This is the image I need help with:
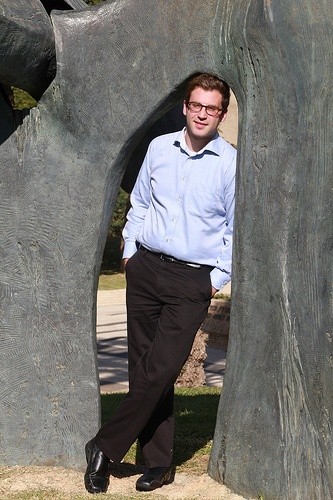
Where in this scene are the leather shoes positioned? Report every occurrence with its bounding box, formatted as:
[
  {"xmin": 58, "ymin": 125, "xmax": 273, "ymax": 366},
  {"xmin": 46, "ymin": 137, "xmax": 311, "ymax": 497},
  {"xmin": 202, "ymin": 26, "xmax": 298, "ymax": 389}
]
[
  {"xmin": 84, "ymin": 437, "xmax": 109, "ymax": 493},
  {"xmin": 136, "ymin": 465, "xmax": 175, "ymax": 491}
]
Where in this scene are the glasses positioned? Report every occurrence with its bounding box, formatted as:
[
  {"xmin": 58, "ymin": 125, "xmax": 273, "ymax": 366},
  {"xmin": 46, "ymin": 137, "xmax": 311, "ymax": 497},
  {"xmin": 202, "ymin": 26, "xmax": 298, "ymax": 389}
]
[{"xmin": 186, "ymin": 101, "xmax": 223, "ymax": 116}]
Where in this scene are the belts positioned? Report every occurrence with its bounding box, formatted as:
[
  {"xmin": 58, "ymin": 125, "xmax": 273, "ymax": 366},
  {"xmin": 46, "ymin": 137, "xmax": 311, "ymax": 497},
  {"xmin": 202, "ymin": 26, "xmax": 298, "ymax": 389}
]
[{"xmin": 137, "ymin": 241, "xmax": 201, "ymax": 269}]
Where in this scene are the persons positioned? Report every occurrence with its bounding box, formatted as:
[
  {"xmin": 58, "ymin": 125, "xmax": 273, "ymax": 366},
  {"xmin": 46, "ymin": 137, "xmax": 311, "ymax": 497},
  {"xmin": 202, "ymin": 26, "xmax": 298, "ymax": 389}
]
[{"xmin": 84, "ymin": 73, "xmax": 236, "ymax": 493}]
